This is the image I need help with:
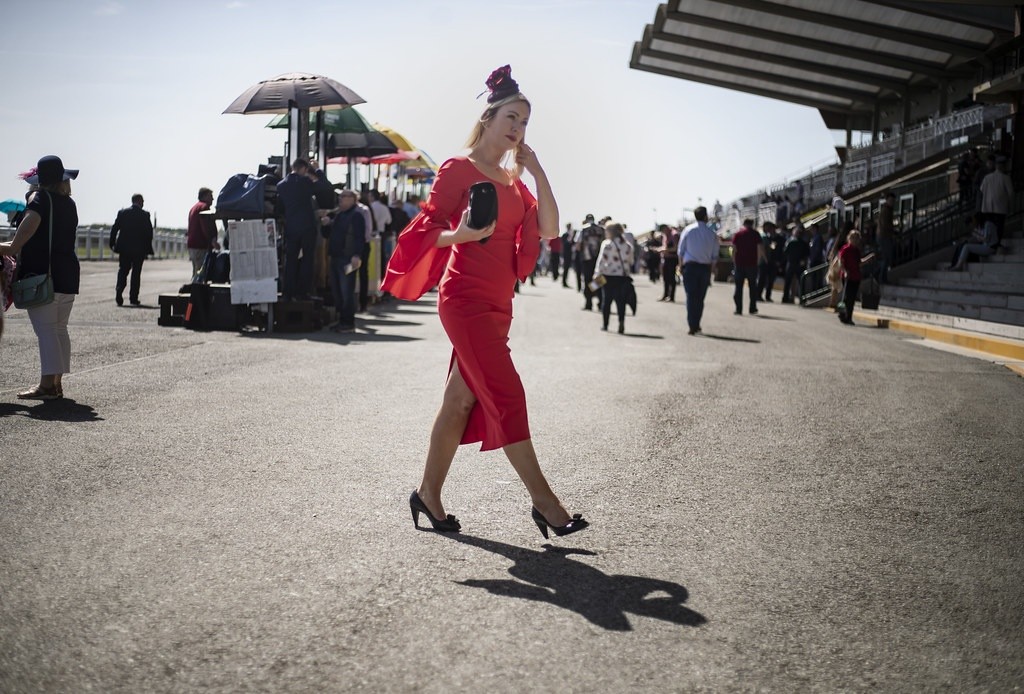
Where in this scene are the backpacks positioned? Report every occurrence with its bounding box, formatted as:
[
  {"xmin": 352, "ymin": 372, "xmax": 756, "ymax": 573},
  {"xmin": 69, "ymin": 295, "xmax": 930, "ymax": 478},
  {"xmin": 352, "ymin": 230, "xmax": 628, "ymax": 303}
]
[
  {"xmin": 216, "ymin": 173, "xmax": 265, "ymax": 214},
  {"xmin": 202, "ymin": 249, "xmax": 230, "ymax": 283}
]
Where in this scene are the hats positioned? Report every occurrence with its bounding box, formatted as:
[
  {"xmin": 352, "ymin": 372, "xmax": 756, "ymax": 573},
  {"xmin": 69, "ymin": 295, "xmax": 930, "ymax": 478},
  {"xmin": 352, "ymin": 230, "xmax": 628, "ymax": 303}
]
[
  {"xmin": 17, "ymin": 155, "xmax": 79, "ymax": 185},
  {"xmin": 582, "ymin": 214, "xmax": 594, "ymax": 225},
  {"xmin": 484, "ymin": 64, "xmax": 528, "ymax": 109}
]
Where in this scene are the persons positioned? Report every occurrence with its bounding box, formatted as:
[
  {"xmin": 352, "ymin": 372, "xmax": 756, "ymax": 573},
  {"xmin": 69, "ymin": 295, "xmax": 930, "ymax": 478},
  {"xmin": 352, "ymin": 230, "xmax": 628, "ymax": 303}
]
[
  {"xmin": 678, "ymin": 205, "xmax": 719, "ymax": 335},
  {"xmin": 732, "ymin": 218, "xmax": 763, "ymax": 317},
  {"xmin": 0, "ymin": 155, "xmax": 80, "ymax": 399},
  {"xmin": 321, "ymin": 190, "xmax": 422, "ymax": 332},
  {"xmin": 620, "ymin": 224, "xmax": 683, "ymax": 303},
  {"xmin": 110, "ymin": 195, "xmax": 154, "ymax": 306},
  {"xmin": 948, "ymin": 147, "xmax": 1012, "ymax": 271},
  {"xmin": 593, "ymin": 220, "xmax": 634, "ymax": 334},
  {"xmin": 514, "ymin": 215, "xmax": 611, "ymax": 310},
  {"xmin": 877, "ymin": 191, "xmax": 899, "ymax": 285},
  {"xmin": 188, "ymin": 187, "xmax": 221, "ymax": 285},
  {"xmin": 380, "ymin": 63, "xmax": 590, "ymax": 539},
  {"xmin": 757, "ymin": 180, "xmax": 845, "ymax": 306},
  {"xmin": 274, "ymin": 160, "xmax": 336, "ymax": 303},
  {"xmin": 838, "ymin": 229, "xmax": 875, "ymax": 325},
  {"xmin": 222, "ymin": 226, "xmax": 229, "ymax": 250}
]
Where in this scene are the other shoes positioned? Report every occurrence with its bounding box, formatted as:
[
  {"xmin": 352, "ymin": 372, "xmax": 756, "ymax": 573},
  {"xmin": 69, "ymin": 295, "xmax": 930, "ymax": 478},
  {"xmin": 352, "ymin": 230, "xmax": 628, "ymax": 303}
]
[
  {"xmin": 56, "ymin": 383, "xmax": 63, "ymax": 397},
  {"xmin": 130, "ymin": 300, "xmax": 140, "ymax": 305},
  {"xmin": 116, "ymin": 287, "xmax": 124, "ymax": 305},
  {"xmin": 766, "ymin": 298, "xmax": 772, "ymax": 302},
  {"xmin": 15, "ymin": 384, "xmax": 58, "ymax": 400},
  {"xmin": 756, "ymin": 297, "xmax": 764, "ymax": 302},
  {"xmin": 330, "ymin": 320, "xmax": 356, "ymax": 335}
]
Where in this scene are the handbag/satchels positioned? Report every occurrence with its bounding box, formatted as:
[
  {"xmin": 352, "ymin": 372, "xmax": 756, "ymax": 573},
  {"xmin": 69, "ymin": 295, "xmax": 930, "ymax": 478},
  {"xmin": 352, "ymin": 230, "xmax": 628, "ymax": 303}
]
[{"xmin": 11, "ymin": 273, "xmax": 55, "ymax": 309}]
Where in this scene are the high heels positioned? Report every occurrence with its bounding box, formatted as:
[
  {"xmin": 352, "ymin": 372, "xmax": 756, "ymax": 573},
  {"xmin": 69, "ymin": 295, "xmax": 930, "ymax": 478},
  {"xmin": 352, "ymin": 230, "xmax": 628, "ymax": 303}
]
[
  {"xmin": 531, "ymin": 504, "xmax": 590, "ymax": 540},
  {"xmin": 409, "ymin": 489, "xmax": 462, "ymax": 532}
]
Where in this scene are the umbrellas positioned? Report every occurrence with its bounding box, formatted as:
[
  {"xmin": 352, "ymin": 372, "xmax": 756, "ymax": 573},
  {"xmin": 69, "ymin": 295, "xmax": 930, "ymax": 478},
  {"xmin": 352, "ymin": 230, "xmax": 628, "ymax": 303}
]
[{"xmin": 223, "ymin": 72, "xmax": 437, "ymax": 203}]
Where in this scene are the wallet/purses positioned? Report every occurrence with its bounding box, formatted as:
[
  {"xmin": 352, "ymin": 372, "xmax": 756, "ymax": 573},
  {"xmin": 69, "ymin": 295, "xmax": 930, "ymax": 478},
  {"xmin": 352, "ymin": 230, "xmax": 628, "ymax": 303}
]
[{"xmin": 467, "ymin": 181, "xmax": 498, "ymax": 243}]
